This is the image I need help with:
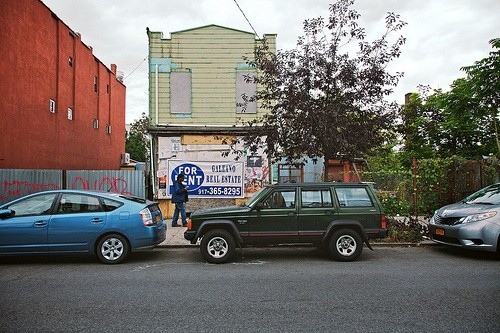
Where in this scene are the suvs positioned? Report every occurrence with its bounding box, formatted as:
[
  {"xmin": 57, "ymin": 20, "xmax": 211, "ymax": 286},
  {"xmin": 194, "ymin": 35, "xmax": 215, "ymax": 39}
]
[{"xmin": 184, "ymin": 180, "xmax": 389, "ymax": 265}]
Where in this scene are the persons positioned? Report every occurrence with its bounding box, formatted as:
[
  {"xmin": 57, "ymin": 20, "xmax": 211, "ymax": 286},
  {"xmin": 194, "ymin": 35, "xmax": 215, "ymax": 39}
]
[{"xmin": 169, "ymin": 173, "xmax": 190, "ymax": 228}]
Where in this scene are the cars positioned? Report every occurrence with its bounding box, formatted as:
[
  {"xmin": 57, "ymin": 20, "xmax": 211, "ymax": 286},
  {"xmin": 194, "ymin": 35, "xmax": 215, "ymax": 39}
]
[
  {"xmin": 429, "ymin": 182, "xmax": 500, "ymax": 254},
  {"xmin": 0, "ymin": 190, "xmax": 168, "ymax": 264}
]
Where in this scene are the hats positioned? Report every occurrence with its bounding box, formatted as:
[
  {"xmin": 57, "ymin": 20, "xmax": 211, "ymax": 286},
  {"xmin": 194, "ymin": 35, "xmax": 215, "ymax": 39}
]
[{"xmin": 178, "ymin": 174, "xmax": 185, "ymax": 178}]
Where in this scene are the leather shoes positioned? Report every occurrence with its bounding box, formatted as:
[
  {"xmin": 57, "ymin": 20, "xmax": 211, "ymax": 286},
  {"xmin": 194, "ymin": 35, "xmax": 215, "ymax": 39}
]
[{"xmin": 172, "ymin": 223, "xmax": 188, "ymax": 227}]
